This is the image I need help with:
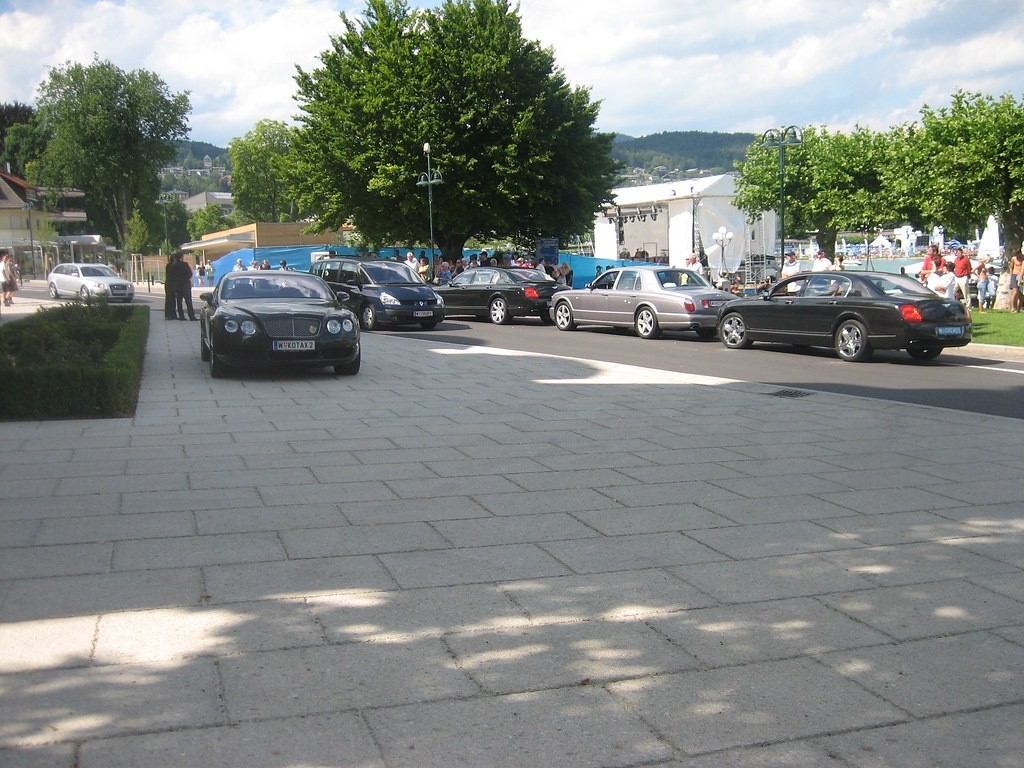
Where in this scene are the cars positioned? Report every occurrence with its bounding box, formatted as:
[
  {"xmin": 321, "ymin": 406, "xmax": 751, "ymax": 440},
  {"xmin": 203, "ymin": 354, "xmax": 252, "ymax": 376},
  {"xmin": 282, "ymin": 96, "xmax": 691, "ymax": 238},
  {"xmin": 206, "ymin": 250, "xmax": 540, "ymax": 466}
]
[
  {"xmin": 714, "ymin": 269, "xmax": 973, "ymax": 364},
  {"xmin": 430, "ymin": 265, "xmax": 572, "ymax": 325},
  {"xmin": 549, "ymin": 266, "xmax": 742, "ymax": 340},
  {"xmin": 48, "ymin": 262, "xmax": 136, "ymax": 303},
  {"xmin": 955, "ymin": 256, "xmax": 1002, "ymax": 301},
  {"xmin": 199, "ymin": 269, "xmax": 361, "ymax": 379}
]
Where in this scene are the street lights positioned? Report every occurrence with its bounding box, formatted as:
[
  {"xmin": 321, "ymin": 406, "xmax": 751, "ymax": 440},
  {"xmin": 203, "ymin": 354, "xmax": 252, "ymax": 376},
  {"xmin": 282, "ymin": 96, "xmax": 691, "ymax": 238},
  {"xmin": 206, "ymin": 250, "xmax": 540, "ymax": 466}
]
[
  {"xmin": 712, "ymin": 226, "xmax": 734, "ymax": 276},
  {"xmin": 23, "ymin": 201, "xmax": 37, "ymax": 278},
  {"xmin": 416, "ymin": 141, "xmax": 446, "ymax": 280},
  {"xmin": 760, "ymin": 125, "xmax": 803, "ymax": 274},
  {"xmin": 155, "ymin": 193, "xmax": 174, "ymax": 262}
]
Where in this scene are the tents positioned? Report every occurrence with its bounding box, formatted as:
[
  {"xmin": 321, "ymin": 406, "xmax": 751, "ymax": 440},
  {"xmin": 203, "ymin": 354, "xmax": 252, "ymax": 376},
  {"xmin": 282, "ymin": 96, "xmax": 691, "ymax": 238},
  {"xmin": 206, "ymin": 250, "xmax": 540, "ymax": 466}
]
[{"xmin": 593, "ymin": 174, "xmax": 775, "ymax": 273}]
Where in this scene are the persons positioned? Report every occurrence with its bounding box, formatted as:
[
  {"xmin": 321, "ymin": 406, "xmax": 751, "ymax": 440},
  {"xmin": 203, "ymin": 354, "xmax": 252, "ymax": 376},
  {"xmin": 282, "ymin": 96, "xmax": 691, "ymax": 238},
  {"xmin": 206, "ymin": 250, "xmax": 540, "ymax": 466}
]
[
  {"xmin": 535, "ymin": 257, "xmax": 574, "ymax": 289},
  {"xmin": 1010, "ymin": 247, "xmax": 1024, "ymax": 313},
  {"xmin": 433, "ymin": 254, "xmax": 469, "ymax": 287},
  {"xmin": 830, "ymin": 255, "xmax": 844, "ymax": 296},
  {"xmin": 813, "ymin": 249, "xmax": 832, "ymax": 271},
  {"xmin": 279, "ymin": 260, "xmax": 288, "ymax": 271},
  {"xmin": 954, "ymin": 247, "xmax": 972, "ymax": 310},
  {"xmin": 232, "ymin": 259, "xmax": 271, "ymax": 272},
  {"xmin": 164, "ymin": 252, "xmax": 201, "ymax": 321},
  {"xmin": 781, "ymin": 252, "xmax": 805, "ymax": 296},
  {"xmin": 595, "ymin": 265, "xmax": 603, "ymax": 278},
  {"xmin": 971, "ymin": 258, "xmax": 998, "ymax": 315},
  {"xmin": 468, "ymin": 250, "xmax": 539, "ymax": 269},
  {"xmin": 606, "ymin": 265, "xmax": 618, "ymax": 280},
  {"xmin": 918, "ymin": 243, "xmax": 956, "ymax": 299},
  {"xmin": 685, "ymin": 253, "xmax": 707, "ymax": 277},
  {"xmin": 390, "ymin": 249, "xmax": 432, "ymax": 283},
  {"xmin": 196, "ymin": 259, "xmax": 216, "ymax": 287},
  {"xmin": 0, "ymin": 253, "xmax": 18, "ymax": 306}
]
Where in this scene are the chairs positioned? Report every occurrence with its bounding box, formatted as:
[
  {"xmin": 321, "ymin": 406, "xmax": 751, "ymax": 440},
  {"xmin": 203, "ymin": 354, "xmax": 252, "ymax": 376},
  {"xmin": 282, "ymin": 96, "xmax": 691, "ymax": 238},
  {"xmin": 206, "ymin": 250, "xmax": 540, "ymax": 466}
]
[{"xmin": 234, "ymin": 283, "xmax": 254, "ymax": 299}]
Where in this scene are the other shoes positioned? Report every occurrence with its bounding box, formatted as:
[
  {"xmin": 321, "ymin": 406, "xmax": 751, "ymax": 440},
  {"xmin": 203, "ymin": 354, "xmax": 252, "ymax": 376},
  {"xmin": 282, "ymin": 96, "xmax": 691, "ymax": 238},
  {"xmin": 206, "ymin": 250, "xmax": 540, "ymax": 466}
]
[
  {"xmin": 3, "ymin": 297, "xmax": 14, "ymax": 306},
  {"xmin": 165, "ymin": 315, "xmax": 200, "ymax": 321}
]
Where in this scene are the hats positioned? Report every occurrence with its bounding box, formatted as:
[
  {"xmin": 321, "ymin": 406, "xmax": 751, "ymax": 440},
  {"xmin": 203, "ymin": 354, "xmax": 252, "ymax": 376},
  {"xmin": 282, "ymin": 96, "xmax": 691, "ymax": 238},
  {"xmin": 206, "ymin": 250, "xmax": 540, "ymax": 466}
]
[
  {"xmin": 479, "ymin": 251, "xmax": 487, "ymax": 255},
  {"xmin": 818, "ymin": 250, "xmax": 825, "ymax": 253},
  {"xmin": 785, "ymin": 251, "xmax": 795, "ymax": 256}
]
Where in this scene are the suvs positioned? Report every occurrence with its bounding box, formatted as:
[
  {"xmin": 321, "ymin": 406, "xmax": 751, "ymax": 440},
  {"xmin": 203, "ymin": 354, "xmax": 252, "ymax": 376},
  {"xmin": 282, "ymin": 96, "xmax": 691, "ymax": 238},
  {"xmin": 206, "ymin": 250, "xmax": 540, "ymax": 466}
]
[{"xmin": 307, "ymin": 254, "xmax": 447, "ymax": 331}]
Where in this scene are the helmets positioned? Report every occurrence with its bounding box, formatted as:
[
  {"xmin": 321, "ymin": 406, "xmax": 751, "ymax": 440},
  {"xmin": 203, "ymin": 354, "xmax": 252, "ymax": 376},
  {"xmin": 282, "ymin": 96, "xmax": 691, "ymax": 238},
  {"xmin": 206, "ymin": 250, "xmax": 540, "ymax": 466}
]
[{"xmin": 280, "ymin": 260, "xmax": 289, "ymax": 264}]
